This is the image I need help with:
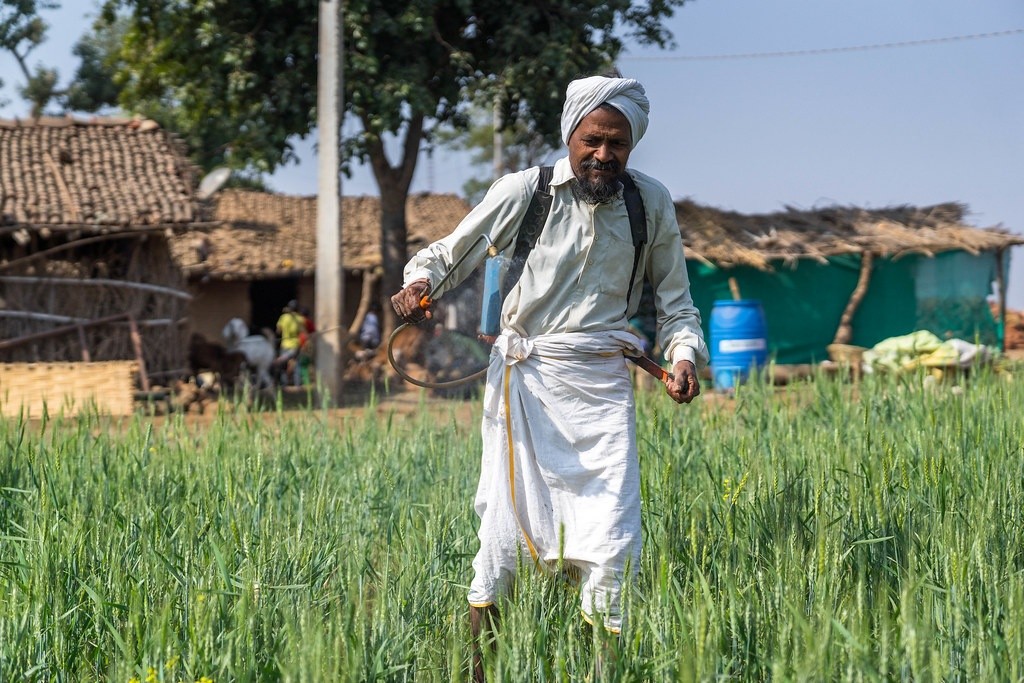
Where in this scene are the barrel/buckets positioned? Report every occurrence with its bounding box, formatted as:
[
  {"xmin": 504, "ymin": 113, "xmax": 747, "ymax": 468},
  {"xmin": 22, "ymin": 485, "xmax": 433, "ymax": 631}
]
[
  {"xmin": 708, "ymin": 298, "xmax": 767, "ymax": 391},
  {"xmin": 476, "ymin": 251, "xmax": 514, "ymax": 343}
]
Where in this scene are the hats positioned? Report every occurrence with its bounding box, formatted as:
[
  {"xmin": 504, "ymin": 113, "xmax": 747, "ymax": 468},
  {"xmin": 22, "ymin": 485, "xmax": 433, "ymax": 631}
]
[{"xmin": 222, "ymin": 318, "xmax": 249, "ymax": 341}]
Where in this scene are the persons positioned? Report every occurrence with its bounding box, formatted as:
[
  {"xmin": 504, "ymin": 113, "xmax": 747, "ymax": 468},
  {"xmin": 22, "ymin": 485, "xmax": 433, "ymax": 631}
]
[
  {"xmin": 188, "ymin": 316, "xmax": 275, "ymax": 413},
  {"xmin": 356, "ymin": 303, "xmax": 382, "ymax": 351},
  {"xmin": 277, "ymin": 299, "xmax": 319, "ymax": 408},
  {"xmin": 389, "ymin": 74, "xmax": 712, "ymax": 683}
]
